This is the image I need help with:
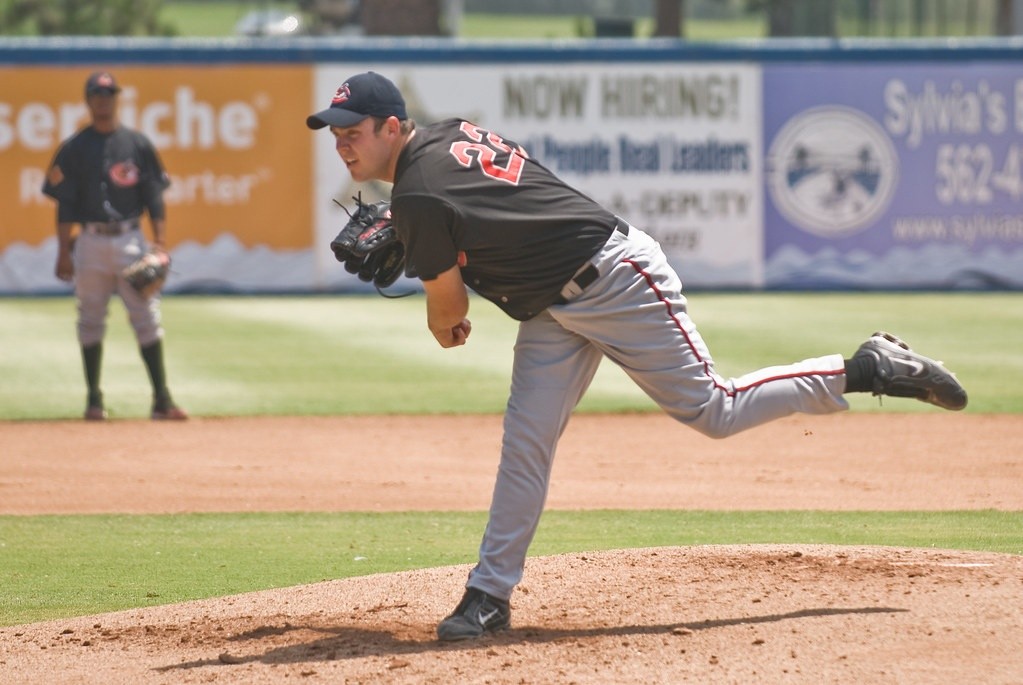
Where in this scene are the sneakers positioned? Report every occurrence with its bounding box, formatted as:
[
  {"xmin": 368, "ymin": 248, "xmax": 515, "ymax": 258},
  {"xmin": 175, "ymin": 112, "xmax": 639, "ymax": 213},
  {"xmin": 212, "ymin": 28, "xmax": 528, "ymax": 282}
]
[
  {"xmin": 436, "ymin": 586, "xmax": 511, "ymax": 641},
  {"xmin": 852, "ymin": 331, "xmax": 968, "ymax": 410}
]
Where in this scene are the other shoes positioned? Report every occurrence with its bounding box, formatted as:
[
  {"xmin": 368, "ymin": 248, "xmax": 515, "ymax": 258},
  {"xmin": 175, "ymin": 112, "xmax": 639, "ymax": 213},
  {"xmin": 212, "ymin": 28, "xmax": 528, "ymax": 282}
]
[
  {"xmin": 85, "ymin": 396, "xmax": 108, "ymax": 420},
  {"xmin": 152, "ymin": 406, "xmax": 190, "ymax": 421}
]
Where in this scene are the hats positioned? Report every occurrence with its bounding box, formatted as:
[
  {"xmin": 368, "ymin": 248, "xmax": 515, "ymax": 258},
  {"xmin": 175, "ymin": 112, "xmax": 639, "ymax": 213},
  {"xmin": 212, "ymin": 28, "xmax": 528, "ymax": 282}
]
[
  {"xmin": 85, "ymin": 72, "xmax": 121, "ymax": 95},
  {"xmin": 306, "ymin": 70, "xmax": 408, "ymax": 130}
]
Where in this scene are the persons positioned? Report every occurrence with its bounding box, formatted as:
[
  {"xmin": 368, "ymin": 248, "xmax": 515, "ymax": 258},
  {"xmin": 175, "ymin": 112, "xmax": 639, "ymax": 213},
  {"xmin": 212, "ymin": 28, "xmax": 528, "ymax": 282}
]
[
  {"xmin": 41, "ymin": 71, "xmax": 187, "ymax": 422},
  {"xmin": 306, "ymin": 71, "xmax": 969, "ymax": 641}
]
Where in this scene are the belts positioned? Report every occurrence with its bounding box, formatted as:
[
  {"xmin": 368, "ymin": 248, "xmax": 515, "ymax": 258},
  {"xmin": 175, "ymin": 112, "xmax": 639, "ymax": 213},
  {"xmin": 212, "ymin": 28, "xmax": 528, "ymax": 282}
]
[
  {"xmin": 553, "ymin": 219, "xmax": 629, "ymax": 305},
  {"xmin": 82, "ymin": 220, "xmax": 140, "ymax": 237}
]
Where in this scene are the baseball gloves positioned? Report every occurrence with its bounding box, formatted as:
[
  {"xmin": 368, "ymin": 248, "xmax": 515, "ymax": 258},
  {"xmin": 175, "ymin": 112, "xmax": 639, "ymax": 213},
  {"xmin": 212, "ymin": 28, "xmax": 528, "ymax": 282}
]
[
  {"xmin": 330, "ymin": 200, "xmax": 407, "ymax": 289},
  {"xmin": 118, "ymin": 250, "xmax": 171, "ymax": 302}
]
